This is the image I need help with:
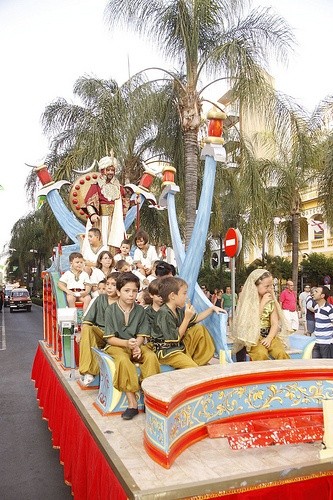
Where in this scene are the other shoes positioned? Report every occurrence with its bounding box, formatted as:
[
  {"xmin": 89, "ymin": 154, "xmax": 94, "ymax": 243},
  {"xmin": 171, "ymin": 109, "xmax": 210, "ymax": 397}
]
[{"xmin": 120, "ymin": 406, "xmax": 138, "ymax": 421}]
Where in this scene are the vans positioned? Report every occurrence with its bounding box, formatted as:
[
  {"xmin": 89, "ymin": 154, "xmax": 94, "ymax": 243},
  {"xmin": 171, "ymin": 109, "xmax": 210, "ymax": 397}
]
[{"xmin": 8, "ymin": 288, "xmax": 32, "ymax": 312}]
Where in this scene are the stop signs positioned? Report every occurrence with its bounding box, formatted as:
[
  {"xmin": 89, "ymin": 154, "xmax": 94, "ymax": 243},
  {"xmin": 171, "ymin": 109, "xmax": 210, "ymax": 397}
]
[{"xmin": 223, "ymin": 227, "xmax": 238, "ymax": 257}]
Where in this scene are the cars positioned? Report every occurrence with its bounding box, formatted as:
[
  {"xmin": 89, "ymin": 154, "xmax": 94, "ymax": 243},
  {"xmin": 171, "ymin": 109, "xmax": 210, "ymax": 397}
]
[{"xmin": 3, "ymin": 285, "xmax": 15, "ymax": 307}]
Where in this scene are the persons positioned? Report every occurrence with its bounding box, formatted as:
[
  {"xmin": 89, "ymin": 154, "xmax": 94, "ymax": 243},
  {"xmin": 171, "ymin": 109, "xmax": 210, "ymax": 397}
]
[
  {"xmin": 148, "ymin": 277, "xmax": 227, "ymax": 369},
  {"xmin": 299, "ymin": 285, "xmax": 311, "ymax": 336},
  {"xmin": 312, "ymin": 286, "xmax": 333, "ymax": 358},
  {"xmin": 81, "ymin": 148, "xmax": 141, "ymax": 258},
  {"xmin": 100, "ymin": 271, "xmax": 161, "ymax": 420},
  {"xmin": 279, "ymin": 281, "xmax": 299, "ymax": 339},
  {"xmin": 229, "ymin": 268, "xmax": 291, "ymax": 361},
  {"xmin": 78, "ymin": 271, "xmax": 121, "ymax": 387},
  {"xmin": 305, "ymin": 286, "xmax": 318, "ymax": 337},
  {"xmin": 201, "ymin": 284, "xmax": 243, "ymax": 331},
  {"xmin": 57, "ymin": 228, "xmax": 177, "ymax": 349},
  {"xmin": 327, "ymin": 289, "xmax": 333, "ymax": 305}
]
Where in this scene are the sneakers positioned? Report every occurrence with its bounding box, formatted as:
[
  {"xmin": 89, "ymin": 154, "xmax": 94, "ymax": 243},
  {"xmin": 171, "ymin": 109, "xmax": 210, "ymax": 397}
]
[{"xmin": 84, "ymin": 373, "xmax": 94, "ymax": 386}]
